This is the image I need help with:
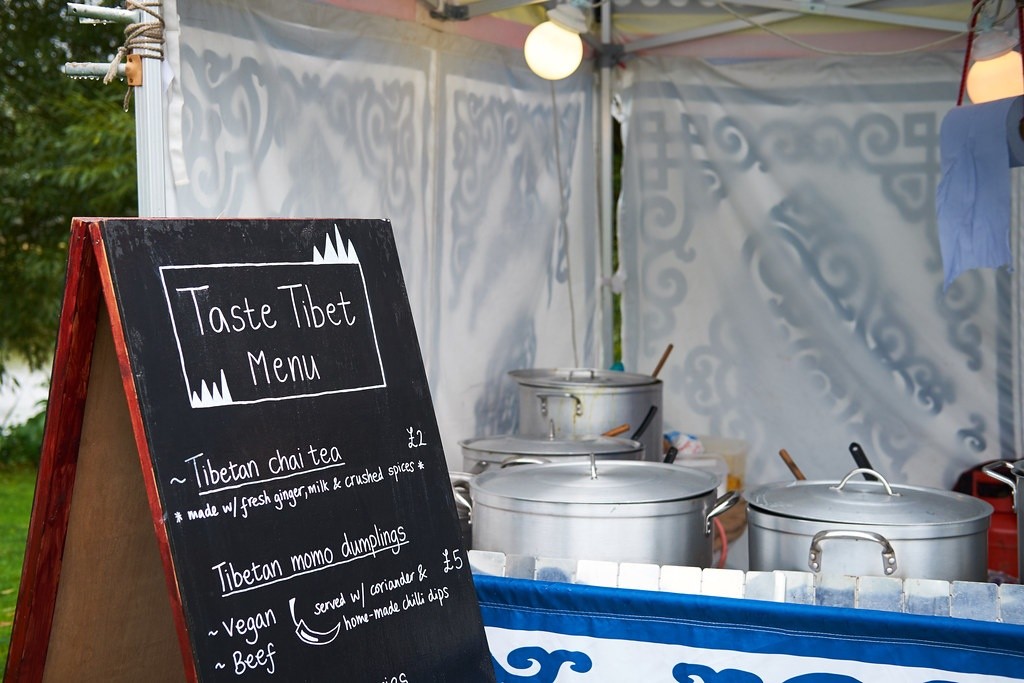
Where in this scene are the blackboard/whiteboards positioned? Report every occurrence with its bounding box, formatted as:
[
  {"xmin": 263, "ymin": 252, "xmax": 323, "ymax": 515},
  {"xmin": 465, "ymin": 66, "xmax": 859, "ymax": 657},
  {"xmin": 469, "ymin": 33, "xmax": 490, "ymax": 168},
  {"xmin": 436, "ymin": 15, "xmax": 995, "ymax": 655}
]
[{"xmin": 1, "ymin": 218, "xmax": 497, "ymax": 683}]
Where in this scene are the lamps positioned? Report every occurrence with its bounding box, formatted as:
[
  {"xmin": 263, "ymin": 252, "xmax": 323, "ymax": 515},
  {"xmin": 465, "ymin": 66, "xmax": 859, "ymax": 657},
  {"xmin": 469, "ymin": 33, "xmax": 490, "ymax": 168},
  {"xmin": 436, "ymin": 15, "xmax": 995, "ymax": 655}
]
[
  {"xmin": 523, "ymin": 0, "xmax": 594, "ymax": 80},
  {"xmin": 963, "ymin": 19, "xmax": 1024, "ymax": 103}
]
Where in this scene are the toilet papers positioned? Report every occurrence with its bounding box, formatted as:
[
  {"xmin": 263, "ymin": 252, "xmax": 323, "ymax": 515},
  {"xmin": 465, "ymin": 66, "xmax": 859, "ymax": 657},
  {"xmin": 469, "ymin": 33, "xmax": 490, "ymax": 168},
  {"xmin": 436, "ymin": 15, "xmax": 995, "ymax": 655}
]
[{"xmin": 933, "ymin": 94, "xmax": 1023, "ymax": 293}]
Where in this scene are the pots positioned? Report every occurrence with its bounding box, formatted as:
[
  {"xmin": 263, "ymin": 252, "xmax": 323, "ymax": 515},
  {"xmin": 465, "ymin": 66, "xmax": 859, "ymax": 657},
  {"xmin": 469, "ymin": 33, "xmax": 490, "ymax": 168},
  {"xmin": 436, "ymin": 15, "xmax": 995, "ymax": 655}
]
[
  {"xmin": 467, "ymin": 464, "xmax": 740, "ymax": 568},
  {"xmin": 507, "ymin": 362, "xmax": 664, "ymax": 468},
  {"xmin": 739, "ymin": 467, "xmax": 994, "ymax": 587},
  {"xmin": 981, "ymin": 458, "xmax": 1024, "ymax": 587},
  {"xmin": 457, "ymin": 435, "xmax": 643, "ymax": 473}
]
